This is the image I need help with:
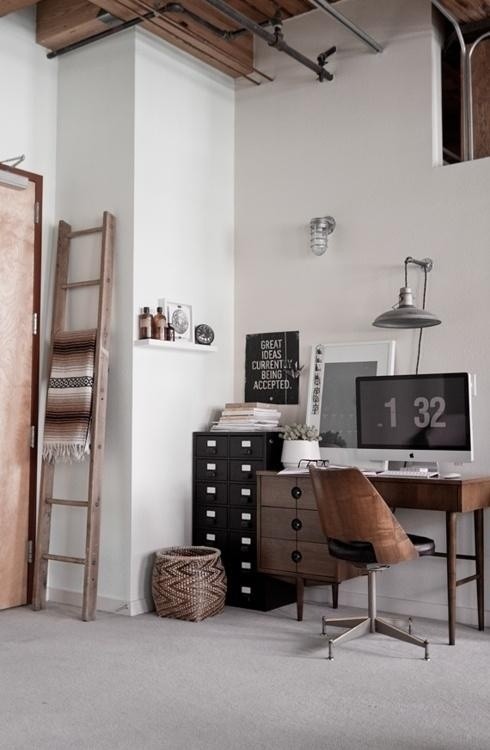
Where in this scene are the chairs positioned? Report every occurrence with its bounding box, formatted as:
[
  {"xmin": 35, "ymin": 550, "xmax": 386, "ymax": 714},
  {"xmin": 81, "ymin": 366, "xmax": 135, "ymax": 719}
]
[{"xmin": 306, "ymin": 465, "xmax": 441, "ymax": 661}]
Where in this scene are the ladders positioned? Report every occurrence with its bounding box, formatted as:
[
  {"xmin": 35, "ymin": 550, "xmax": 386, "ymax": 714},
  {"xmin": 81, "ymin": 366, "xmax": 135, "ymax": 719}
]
[{"xmin": 29, "ymin": 212, "xmax": 118, "ymax": 621}]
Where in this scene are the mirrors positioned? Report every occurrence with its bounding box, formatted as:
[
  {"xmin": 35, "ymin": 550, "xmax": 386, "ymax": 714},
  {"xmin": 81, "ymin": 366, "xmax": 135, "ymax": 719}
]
[{"xmin": 301, "ymin": 341, "xmax": 396, "ymax": 471}]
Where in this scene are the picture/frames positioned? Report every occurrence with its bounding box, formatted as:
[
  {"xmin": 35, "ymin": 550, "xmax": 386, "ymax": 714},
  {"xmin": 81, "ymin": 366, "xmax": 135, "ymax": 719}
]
[{"xmin": 160, "ymin": 301, "xmax": 194, "ymax": 342}]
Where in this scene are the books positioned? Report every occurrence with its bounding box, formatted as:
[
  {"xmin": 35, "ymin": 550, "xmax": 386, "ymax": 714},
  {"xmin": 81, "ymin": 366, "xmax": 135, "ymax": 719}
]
[
  {"xmin": 210, "ymin": 402, "xmax": 281, "ymax": 432},
  {"xmin": 158, "ymin": 297, "xmax": 195, "ymax": 343}
]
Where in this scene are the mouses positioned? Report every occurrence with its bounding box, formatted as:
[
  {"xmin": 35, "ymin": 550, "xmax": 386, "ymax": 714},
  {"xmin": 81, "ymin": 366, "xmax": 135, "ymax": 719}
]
[{"xmin": 444, "ymin": 473, "xmax": 462, "ymax": 479}]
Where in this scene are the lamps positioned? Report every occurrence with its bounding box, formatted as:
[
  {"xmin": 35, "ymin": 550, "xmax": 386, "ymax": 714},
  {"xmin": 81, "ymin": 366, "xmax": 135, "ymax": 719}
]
[
  {"xmin": 309, "ymin": 215, "xmax": 334, "ymax": 256},
  {"xmin": 370, "ymin": 254, "xmax": 444, "ymax": 330}
]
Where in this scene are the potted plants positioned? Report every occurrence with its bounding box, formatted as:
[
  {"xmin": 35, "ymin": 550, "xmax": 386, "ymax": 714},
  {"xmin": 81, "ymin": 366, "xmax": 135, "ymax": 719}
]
[{"xmin": 278, "ymin": 422, "xmax": 323, "ymax": 468}]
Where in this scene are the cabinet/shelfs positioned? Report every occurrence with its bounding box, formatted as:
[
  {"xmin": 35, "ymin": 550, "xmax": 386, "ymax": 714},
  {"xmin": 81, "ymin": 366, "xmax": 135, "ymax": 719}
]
[{"xmin": 191, "ymin": 429, "xmax": 298, "ymax": 613}]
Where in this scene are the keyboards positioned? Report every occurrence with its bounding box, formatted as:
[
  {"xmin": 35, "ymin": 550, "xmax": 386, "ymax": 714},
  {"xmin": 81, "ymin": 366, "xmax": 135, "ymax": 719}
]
[{"xmin": 363, "ymin": 472, "xmax": 440, "ymax": 478}]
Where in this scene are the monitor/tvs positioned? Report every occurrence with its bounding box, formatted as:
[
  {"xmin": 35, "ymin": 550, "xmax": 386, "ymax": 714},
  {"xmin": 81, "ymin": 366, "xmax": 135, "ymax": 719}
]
[{"xmin": 357, "ymin": 374, "xmax": 474, "ymax": 472}]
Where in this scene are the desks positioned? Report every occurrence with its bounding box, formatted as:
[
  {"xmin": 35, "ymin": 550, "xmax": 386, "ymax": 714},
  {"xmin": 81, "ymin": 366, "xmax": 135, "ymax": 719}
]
[{"xmin": 253, "ymin": 462, "xmax": 489, "ymax": 646}]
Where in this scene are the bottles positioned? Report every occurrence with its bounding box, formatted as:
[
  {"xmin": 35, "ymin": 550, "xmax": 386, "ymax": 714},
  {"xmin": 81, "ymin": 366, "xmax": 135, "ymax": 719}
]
[{"xmin": 139, "ymin": 307, "xmax": 175, "ymax": 340}]
